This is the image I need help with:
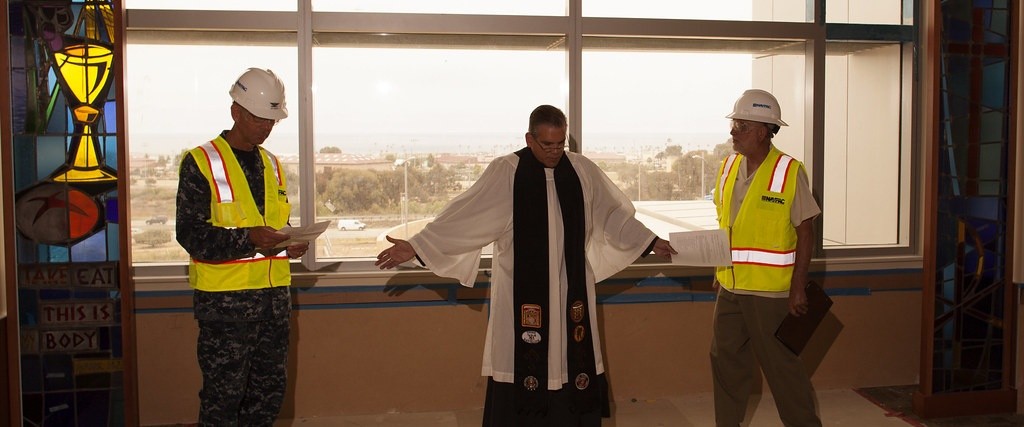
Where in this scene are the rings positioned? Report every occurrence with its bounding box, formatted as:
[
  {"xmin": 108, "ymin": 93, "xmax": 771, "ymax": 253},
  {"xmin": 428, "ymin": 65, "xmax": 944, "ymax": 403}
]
[{"xmin": 300, "ymin": 251, "xmax": 302, "ymax": 255}]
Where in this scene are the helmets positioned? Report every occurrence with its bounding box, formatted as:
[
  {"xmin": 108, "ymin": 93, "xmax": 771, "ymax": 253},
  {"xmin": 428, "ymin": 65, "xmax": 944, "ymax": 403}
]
[
  {"xmin": 229, "ymin": 68, "xmax": 289, "ymax": 120},
  {"xmin": 725, "ymin": 90, "xmax": 788, "ymax": 127}
]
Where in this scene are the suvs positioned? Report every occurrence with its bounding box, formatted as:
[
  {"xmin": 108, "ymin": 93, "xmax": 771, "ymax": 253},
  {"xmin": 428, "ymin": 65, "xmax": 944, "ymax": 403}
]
[{"xmin": 146, "ymin": 216, "xmax": 168, "ymax": 226}]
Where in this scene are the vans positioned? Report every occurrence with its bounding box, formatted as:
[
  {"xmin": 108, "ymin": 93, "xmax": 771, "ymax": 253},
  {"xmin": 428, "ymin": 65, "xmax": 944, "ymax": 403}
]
[{"xmin": 338, "ymin": 220, "xmax": 366, "ymax": 231}]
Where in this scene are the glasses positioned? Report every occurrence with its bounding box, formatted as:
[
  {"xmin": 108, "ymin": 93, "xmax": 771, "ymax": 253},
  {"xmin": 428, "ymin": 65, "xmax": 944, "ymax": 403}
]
[
  {"xmin": 239, "ymin": 105, "xmax": 277, "ymax": 126},
  {"xmin": 530, "ymin": 132, "xmax": 570, "ymax": 153},
  {"xmin": 731, "ymin": 121, "xmax": 762, "ymax": 132}
]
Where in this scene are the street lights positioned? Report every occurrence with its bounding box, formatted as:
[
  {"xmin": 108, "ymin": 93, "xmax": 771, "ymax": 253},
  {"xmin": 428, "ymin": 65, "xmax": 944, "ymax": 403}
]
[
  {"xmin": 692, "ymin": 155, "xmax": 704, "ymax": 200},
  {"xmin": 395, "ymin": 159, "xmax": 409, "ymax": 242}
]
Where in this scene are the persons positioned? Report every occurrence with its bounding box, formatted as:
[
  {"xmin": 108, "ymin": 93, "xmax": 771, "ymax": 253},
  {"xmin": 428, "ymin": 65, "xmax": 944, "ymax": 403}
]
[
  {"xmin": 175, "ymin": 65, "xmax": 309, "ymax": 427},
  {"xmin": 374, "ymin": 104, "xmax": 678, "ymax": 427},
  {"xmin": 709, "ymin": 89, "xmax": 823, "ymax": 427}
]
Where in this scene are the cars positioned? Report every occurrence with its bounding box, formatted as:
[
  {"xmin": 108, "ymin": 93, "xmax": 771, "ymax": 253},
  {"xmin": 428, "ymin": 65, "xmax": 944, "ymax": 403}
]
[{"xmin": 705, "ymin": 196, "xmax": 712, "ymax": 200}]
